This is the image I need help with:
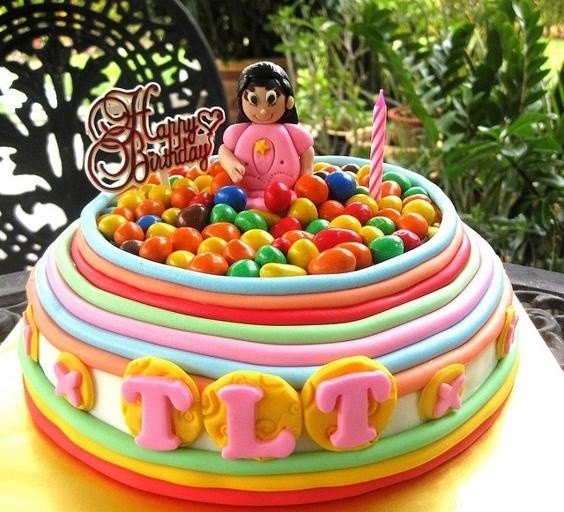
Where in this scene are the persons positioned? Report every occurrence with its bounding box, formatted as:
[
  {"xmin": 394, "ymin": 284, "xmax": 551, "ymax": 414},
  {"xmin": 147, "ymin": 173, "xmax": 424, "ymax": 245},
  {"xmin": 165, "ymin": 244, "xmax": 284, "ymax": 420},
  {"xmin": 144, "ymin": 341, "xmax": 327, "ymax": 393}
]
[{"xmin": 218, "ymin": 61, "xmax": 314, "ymax": 191}]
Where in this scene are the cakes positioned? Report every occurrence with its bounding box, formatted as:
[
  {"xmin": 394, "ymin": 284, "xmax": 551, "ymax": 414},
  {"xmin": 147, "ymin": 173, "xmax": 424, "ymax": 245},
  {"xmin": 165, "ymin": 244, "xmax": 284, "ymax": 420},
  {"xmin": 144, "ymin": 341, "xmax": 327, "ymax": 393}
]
[{"xmin": 18, "ymin": 60, "xmax": 520, "ymax": 508}]
